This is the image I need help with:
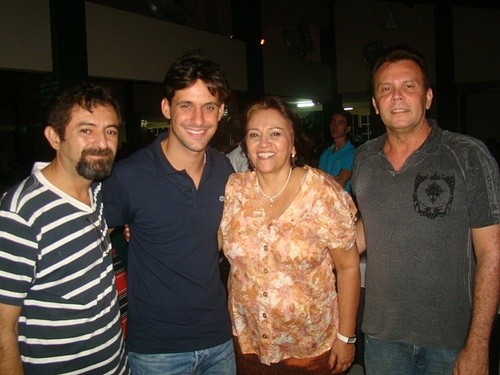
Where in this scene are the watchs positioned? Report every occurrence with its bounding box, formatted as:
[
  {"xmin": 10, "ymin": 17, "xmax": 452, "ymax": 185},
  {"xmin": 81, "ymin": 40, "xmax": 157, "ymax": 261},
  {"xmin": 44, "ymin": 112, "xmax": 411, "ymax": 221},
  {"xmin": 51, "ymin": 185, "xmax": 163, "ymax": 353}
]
[{"xmin": 336, "ymin": 331, "xmax": 357, "ymax": 345}]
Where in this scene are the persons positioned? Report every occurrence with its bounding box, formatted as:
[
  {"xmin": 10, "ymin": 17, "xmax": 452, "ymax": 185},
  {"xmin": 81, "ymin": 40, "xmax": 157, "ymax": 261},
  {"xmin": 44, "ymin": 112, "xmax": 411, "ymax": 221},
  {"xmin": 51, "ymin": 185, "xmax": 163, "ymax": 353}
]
[
  {"xmin": 0, "ymin": 80, "xmax": 132, "ymax": 375},
  {"xmin": 100, "ymin": 49, "xmax": 359, "ymax": 375},
  {"xmin": 350, "ymin": 50, "xmax": 500, "ymax": 375}
]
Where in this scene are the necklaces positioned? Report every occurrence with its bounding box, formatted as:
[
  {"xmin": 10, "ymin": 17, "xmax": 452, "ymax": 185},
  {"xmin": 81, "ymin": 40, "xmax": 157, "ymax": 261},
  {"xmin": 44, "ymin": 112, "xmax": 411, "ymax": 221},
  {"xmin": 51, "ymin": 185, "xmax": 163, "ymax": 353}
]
[{"xmin": 256, "ymin": 166, "xmax": 293, "ymax": 209}]
